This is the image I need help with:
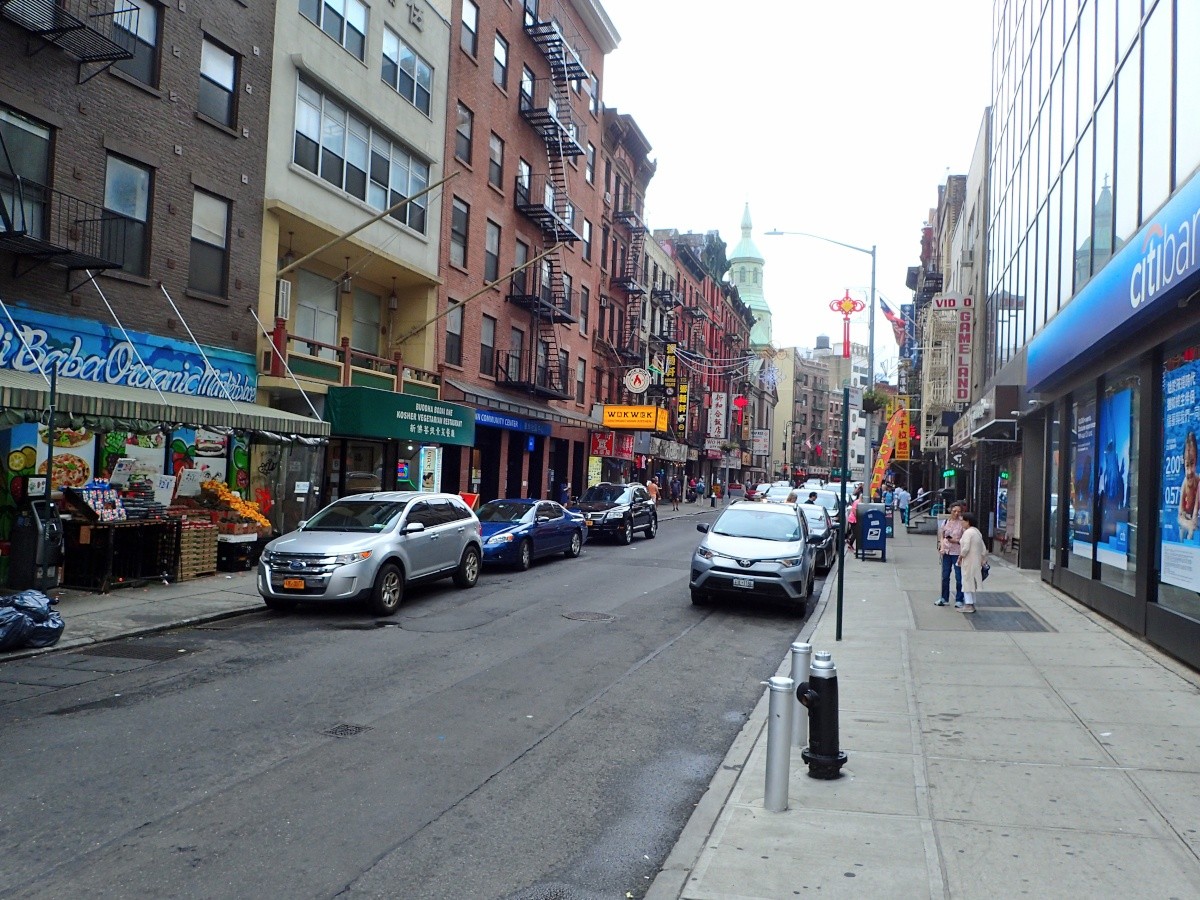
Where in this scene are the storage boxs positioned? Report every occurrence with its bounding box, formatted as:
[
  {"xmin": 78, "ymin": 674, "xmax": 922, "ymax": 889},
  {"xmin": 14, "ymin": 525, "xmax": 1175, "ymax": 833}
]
[{"xmin": 210, "ymin": 510, "xmax": 271, "ymax": 543}]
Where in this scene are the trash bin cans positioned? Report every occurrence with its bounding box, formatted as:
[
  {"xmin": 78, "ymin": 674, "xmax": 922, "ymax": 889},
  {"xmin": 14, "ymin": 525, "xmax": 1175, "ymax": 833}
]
[
  {"xmin": 885, "ymin": 503, "xmax": 895, "ymax": 538},
  {"xmin": 931, "ymin": 504, "xmax": 943, "ymax": 516},
  {"xmin": 856, "ymin": 502, "xmax": 887, "ymax": 563},
  {"xmin": 6, "ymin": 499, "xmax": 65, "ymax": 591}
]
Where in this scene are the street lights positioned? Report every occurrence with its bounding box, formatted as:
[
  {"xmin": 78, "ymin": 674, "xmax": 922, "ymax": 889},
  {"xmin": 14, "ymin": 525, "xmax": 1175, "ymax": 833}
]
[{"xmin": 763, "ymin": 229, "xmax": 877, "ymax": 504}]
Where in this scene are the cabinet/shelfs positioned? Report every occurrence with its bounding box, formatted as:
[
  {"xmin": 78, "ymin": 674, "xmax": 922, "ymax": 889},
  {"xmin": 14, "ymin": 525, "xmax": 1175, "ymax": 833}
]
[{"xmin": 60, "ymin": 520, "xmax": 180, "ymax": 591}]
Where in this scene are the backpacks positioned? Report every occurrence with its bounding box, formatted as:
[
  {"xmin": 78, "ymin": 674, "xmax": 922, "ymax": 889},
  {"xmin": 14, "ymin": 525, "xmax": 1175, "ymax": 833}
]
[{"xmin": 697, "ymin": 483, "xmax": 704, "ymax": 494}]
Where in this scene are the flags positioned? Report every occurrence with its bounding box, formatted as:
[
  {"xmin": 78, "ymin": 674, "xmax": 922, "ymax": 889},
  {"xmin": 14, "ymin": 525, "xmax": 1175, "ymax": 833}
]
[
  {"xmin": 806, "ymin": 440, "xmax": 823, "ymax": 456},
  {"xmin": 880, "ymin": 299, "xmax": 906, "ymax": 346}
]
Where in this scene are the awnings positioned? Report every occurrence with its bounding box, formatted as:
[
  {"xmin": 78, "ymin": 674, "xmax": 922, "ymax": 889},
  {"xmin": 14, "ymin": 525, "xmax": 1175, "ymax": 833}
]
[{"xmin": 0, "ymin": 367, "xmax": 330, "ymax": 438}]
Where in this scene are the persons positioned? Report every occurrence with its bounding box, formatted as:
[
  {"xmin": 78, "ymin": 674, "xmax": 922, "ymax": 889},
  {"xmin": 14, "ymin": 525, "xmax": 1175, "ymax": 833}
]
[
  {"xmin": 933, "ymin": 502, "xmax": 987, "ymax": 613},
  {"xmin": 559, "ymin": 478, "xmax": 569, "ymax": 508},
  {"xmin": 645, "ymin": 475, "xmax": 818, "ymax": 512},
  {"xmin": 1178, "ymin": 432, "xmax": 1200, "ymax": 543},
  {"xmin": 846, "ymin": 480, "xmax": 925, "ymax": 551}
]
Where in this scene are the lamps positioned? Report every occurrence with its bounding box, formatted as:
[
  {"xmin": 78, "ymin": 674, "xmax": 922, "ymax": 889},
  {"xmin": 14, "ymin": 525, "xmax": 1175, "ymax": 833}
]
[
  {"xmin": 341, "ymin": 256, "xmax": 351, "ymax": 293},
  {"xmin": 388, "ymin": 277, "xmax": 398, "ymax": 310},
  {"xmin": 286, "ymin": 232, "xmax": 294, "ymax": 273}
]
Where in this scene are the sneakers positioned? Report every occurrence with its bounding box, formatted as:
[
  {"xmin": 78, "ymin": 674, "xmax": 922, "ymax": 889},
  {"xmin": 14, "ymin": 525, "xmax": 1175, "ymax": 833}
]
[
  {"xmin": 955, "ymin": 601, "xmax": 963, "ymax": 607},
  {"xmin": 934, "ymin": 598, "xmax": 950, "ymax": 605}
]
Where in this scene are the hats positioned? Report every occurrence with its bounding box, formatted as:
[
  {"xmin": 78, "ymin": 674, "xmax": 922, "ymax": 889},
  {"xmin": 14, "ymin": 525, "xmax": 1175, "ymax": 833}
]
[{"xmin": 699, "ymin": 478, "xmax": 703, "ymax": 482}]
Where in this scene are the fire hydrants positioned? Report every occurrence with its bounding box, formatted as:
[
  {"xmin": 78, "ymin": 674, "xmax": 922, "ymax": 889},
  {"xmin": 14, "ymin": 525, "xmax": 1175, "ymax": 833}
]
[{"xmin": 796, "ymin": 648, "xmax": 847, "ymax": 781}]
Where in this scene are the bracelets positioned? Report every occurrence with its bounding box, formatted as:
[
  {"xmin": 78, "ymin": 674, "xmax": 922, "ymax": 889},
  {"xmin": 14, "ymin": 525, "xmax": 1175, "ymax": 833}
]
[{"xmin": 957, "ymin": 541, "xmax": 960, "ymax": 544}]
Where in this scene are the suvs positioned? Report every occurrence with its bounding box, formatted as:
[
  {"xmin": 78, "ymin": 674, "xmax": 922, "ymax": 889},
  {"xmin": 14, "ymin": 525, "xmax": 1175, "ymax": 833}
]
[
  {"xmin": 257, "ymin": 490, "xmax": 484, "ymax": 616},
  {"xmin": 572, "ymin": 482, "xmax": 659, "ymax": 546},
  {"xmin": 689, "ymin": 497, "xmax": 825, "ymax": 618}
]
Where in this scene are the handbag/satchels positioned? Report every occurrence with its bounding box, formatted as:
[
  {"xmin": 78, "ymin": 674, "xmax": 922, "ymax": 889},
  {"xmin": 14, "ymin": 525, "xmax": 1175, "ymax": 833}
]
[
  {"xmin": 981, "ymin": 562, "xmax": 990, "ymax": 582},
  {"xmin": 938, "ymin": 519, "xmax": 948, "ymax": 565}
]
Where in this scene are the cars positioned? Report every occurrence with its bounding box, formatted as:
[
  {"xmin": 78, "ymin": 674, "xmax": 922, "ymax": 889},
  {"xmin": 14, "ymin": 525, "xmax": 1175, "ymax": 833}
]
[
  {"xmin": 473, "ymin": 499, "xmax": 589, "ymax": 573},
  {"xmin": 798, "ymin": 504, "xmax": 842, "ymax": 573},
  {"xmin": 743, "ymin": 478, "xmax": 864, "ymax": 543}
]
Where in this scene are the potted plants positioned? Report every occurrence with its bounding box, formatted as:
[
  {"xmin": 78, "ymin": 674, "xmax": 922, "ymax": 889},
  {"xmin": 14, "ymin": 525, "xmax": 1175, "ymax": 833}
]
[{"xmin": 863, "ymin": 391, "xmax": 891, "ymax": 414}]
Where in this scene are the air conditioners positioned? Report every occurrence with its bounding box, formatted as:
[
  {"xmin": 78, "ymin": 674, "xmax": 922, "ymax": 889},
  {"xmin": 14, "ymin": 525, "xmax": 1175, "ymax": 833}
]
[
  {"xmin": 275, "ymin": 279, "xmax": 292, "ymax": 320},
  {"xmin": 959, "ymin": 250, "xmax": 973, "ymax": 267}
]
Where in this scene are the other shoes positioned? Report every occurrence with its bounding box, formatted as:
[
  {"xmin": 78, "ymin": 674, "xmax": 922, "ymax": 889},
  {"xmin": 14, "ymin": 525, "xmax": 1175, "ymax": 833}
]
[
  {"xmin": 672, "ymin": 507, "xmax": 680, "ymax": 511},
  {"xmin": 956, "ymin": 606, "xmax": 975, "ymax": 613},
  {"xmin": 847, "ymin": 545, "xmax": 854, "ymax": 550}
]
